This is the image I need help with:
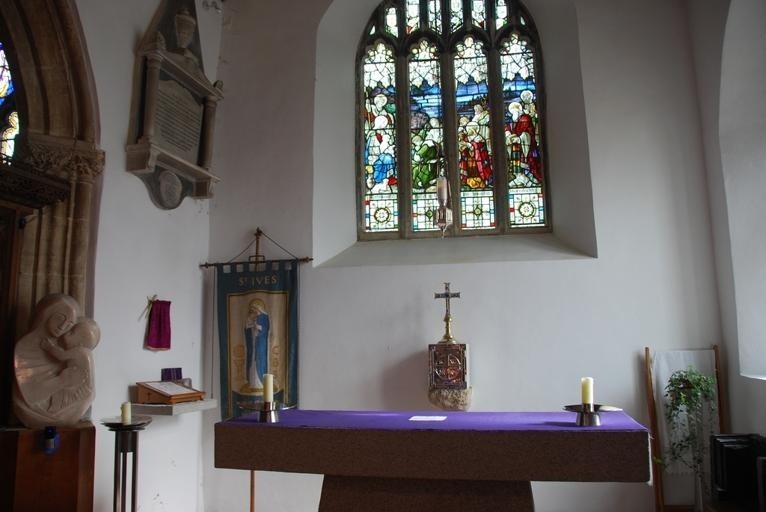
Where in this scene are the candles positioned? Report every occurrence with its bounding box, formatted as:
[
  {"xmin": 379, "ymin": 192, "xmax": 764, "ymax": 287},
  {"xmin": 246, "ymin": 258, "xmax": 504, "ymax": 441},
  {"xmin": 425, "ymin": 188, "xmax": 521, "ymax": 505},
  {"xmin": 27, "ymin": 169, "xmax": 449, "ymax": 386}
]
[
  {"xmin": 580, "ymin": 377, "xmax": 595, "ymax": 404},
  {"xmin": 262, "ymin": 374, "xmax": 273, "ymax": 402},
  {"xmin": 436, "ymin": 174, "xmax": 448, "ymax": 207},
  {"xmin": 121, "ymin": 402, "xmax": 133, "ymax": 423}
]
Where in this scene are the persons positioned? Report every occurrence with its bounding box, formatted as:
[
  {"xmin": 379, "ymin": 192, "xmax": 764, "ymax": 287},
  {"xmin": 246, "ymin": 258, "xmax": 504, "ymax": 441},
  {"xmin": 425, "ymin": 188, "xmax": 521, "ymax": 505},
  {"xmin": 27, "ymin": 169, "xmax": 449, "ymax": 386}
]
[
  {"xmin": 11, "ymin": 295, "xmax": 100, "ymax": 426},
  {"xmin": 245, "ymin": 298, "xmax": 270, "ymax": 390}
]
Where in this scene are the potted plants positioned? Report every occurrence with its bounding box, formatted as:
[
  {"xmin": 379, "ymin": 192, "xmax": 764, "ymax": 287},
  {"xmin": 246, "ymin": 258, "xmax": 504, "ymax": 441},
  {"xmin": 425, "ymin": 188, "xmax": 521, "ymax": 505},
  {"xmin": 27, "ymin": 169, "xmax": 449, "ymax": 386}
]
[{"xmin": 655, "ymin": 367, "xmax": 722, "ymax": 512}]
